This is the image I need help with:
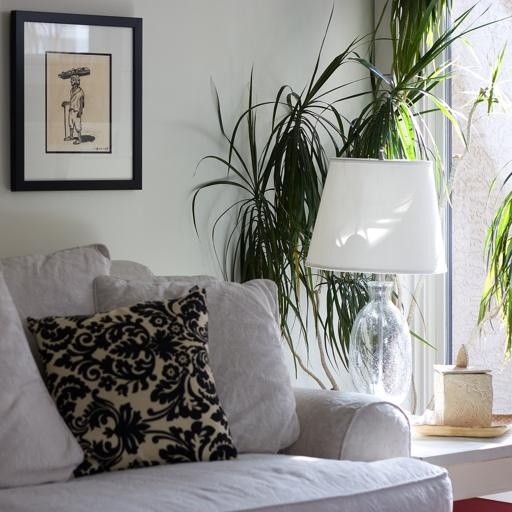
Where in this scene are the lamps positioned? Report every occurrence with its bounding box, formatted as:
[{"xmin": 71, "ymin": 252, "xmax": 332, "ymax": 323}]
[{"xmin": 304, "ymin": 149, "xmax": 449, "ymax": 405}]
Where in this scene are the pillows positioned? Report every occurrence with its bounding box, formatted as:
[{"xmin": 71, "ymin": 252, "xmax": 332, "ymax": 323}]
[
  {"xmin": 22, "ymin": 283, "xmax": 241, "ymax": 478},
  {"xmin": 1, "ymin": 241, "xmax": 113, "ymax": 324},
  {"xmin": 91, "ymin": 271, "xmax": 300, "ymax": 458},
  {"xmin": 0, "ymin": 263, "xmax": 83, "ymax": 493}
]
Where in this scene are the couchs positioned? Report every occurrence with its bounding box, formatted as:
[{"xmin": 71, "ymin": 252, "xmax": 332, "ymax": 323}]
[{"xmin": 0, "ymin": 259, "xmax": 454, "ymax": 511}]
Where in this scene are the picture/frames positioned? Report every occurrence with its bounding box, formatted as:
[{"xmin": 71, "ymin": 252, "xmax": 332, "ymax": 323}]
[{"xmin": 10, "ymin": 8, "xmax": 142, "ymax": 192}]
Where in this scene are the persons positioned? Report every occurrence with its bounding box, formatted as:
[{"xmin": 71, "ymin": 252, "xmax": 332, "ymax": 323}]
[{"xmin": 61, "ymin": 74, "xmax": 84, "ymax": 144}]
